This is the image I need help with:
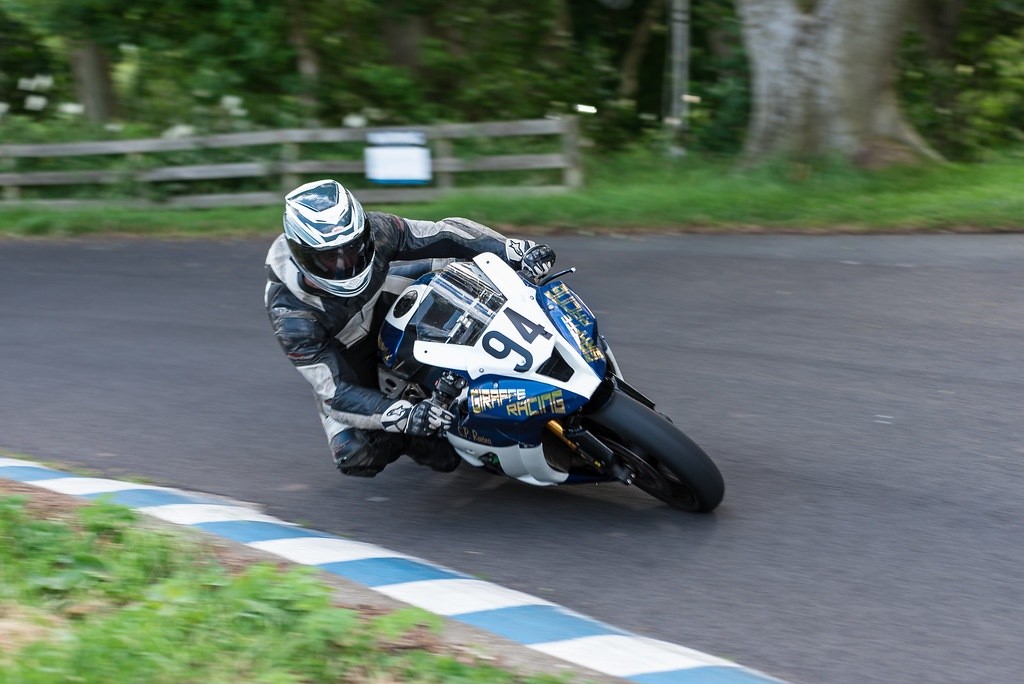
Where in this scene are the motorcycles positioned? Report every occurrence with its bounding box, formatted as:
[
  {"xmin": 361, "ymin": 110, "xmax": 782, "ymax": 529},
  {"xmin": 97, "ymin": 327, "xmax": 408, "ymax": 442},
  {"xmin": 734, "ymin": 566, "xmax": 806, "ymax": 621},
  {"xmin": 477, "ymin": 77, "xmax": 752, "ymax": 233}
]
[{"xmin": 377, "ymin": 252, "xmax": 725, "ymax": 514}]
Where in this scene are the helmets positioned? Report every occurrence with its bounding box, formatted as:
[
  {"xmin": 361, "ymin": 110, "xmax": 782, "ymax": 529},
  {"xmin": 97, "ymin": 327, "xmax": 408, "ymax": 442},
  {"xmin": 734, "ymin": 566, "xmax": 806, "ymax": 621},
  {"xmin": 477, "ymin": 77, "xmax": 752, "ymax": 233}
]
[{"xmin": 282, "ymin": 179, "xmax": 376, "ymax": 298}]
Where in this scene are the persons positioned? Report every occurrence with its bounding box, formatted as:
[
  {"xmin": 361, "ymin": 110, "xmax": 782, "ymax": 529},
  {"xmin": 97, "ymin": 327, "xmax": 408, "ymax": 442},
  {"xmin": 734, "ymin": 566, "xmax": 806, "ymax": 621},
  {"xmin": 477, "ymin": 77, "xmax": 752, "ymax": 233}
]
[{"xmin": 261, "ymin": 179, "xmax": 556, "ymax": 479}]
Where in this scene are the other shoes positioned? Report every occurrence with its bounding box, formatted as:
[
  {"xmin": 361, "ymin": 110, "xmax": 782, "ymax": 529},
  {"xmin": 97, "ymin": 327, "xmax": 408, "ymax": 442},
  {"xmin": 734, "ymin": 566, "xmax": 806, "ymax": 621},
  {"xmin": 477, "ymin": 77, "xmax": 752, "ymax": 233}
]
[{"xmin": 405, "ymin": 439, "xmax": 460, "ymax": 473}]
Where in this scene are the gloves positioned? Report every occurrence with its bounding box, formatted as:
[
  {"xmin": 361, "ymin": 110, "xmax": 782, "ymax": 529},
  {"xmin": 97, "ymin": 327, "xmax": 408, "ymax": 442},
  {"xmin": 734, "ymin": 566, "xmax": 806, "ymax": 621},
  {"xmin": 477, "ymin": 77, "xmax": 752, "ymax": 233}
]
[
  {"xmin": 506, "ymin": 238, "xmax": 556, "ymax": 279},
  {"xmin": 379, "ymin": 400, "xmax": 455, "ymax": 437}
]
